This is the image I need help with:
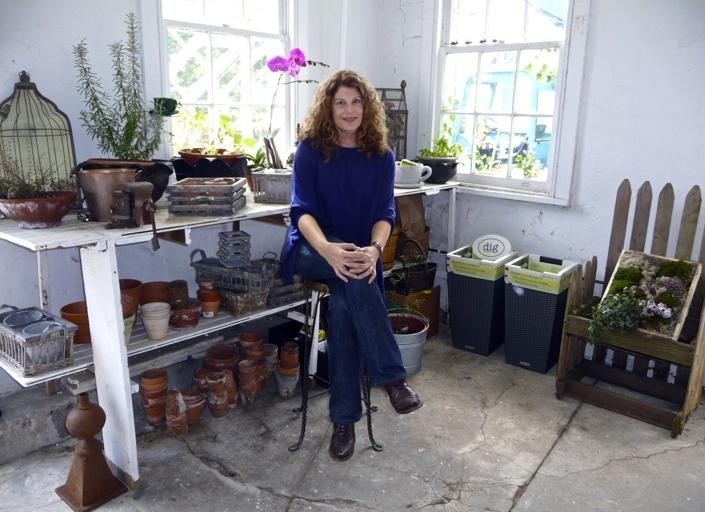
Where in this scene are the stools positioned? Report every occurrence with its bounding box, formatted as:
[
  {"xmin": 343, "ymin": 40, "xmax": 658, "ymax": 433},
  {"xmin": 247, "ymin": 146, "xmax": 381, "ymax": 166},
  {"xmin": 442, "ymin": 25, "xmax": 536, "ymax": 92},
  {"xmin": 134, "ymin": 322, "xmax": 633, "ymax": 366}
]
[{"xmin": 290, "ymin": 278, "xmax": 385, "ymax": 452}]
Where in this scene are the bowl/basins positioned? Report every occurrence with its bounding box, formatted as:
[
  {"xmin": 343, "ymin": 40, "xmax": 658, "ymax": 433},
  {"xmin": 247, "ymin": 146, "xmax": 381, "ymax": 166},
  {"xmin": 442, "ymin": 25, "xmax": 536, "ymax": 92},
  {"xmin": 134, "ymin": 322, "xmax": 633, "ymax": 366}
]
[{"xmin": 63, "ymin": 283, "xmax": 200, "ymax": 340}]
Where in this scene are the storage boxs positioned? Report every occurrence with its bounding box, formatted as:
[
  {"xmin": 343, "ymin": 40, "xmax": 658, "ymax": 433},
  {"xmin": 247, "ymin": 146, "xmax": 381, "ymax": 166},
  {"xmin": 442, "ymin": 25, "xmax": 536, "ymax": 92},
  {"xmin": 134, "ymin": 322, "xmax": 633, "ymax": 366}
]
[{"xmin": 389, "ymin": 285, "xmax": 444, "ymax": 319}]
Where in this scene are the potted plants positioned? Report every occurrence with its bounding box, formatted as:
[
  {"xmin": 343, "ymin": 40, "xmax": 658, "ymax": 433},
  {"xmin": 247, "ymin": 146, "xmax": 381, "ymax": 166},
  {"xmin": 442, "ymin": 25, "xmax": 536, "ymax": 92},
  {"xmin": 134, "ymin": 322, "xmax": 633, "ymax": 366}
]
[
  {"xmin": 178, "ymin": 110, "xmax": 246, "ymax": 178},
  {"xmin": 243, "ymin": 147, "xmax": 266, "ymax": 192},
  {"xmin": 416, "ymin": 94, "xmax": 463, "ymax": 186},
  {"xmin": 0, "ymin": 143, "xmax": 75, "ymax": 230},
  {"xmin": 386, "ymin": 253, "xmax": 429, "ymax": 375},
  {"xmin": 390, "ymin": 253, "xmax": 438, "ymax": 295},
  {"xmin": 71, "ymin": 8, "xmax": 173, "ymax": 223}
]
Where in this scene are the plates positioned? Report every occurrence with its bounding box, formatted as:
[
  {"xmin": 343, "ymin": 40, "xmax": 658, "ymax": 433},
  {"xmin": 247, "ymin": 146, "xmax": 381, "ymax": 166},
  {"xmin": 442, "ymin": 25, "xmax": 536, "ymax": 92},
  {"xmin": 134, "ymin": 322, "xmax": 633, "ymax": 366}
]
[{"xmin": 394, "ymin": 182, "xmax": 425, "ymax": 189}]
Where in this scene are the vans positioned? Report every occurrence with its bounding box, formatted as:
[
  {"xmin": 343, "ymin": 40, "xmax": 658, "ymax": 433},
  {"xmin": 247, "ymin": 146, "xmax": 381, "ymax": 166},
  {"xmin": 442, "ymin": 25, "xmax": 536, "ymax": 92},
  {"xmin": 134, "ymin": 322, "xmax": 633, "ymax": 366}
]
[{"xmin": 451, "ymin": 70, "xmax": 550, "ymax": 170}]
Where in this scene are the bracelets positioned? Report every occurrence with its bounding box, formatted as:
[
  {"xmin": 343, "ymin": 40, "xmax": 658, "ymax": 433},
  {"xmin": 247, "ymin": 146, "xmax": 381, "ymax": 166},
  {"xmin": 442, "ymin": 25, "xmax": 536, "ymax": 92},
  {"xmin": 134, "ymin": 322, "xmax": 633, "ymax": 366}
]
[{"xmin": 371, "ymin": 240, "xmax": 384, "ymax": 255}]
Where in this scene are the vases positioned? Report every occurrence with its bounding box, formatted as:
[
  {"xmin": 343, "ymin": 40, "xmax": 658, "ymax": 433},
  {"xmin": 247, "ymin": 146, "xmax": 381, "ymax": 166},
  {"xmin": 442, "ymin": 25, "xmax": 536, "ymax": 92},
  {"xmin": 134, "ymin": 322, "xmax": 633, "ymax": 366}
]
[
  {"xmin": 0, "ymin": 275, "xmax": 300, "ymax": 437},
  {"xmin": 249, "ymin": 168, "xmax": 292, "ymax": 203},
  {"xmin": 381, "ymin": 225, "xmax": 400, "ymax": 269},
  {"xmin": 597, "ymin": 249, "xmax": 703, "ymax": 343}
]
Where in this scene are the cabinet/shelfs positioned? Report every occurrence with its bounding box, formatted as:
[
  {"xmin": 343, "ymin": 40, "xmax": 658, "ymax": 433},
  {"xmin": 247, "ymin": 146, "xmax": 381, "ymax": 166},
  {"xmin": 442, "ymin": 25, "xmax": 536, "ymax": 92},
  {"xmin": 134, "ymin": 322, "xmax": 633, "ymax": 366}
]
[{"xmin": 0, "ymin": 181, "xmax": 462, "ymax": 491}]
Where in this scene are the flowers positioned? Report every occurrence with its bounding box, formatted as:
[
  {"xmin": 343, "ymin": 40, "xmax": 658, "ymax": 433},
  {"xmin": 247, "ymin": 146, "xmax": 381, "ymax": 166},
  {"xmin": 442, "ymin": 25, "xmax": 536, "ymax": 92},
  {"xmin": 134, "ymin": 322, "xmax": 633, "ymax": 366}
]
[
  {"xmin": 265, "ymin": 48, "xmax": 330, "ymax": 169},
  {"xmin": 586, "ymin": 252, "xmax": 697, "ymax": 346}
]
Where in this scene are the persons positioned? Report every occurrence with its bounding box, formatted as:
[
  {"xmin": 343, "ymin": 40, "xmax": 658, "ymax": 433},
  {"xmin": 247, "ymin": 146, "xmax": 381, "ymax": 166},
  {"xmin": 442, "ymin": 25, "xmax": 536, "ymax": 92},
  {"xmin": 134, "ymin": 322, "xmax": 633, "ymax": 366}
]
[{"xmin": 278, "ymin": 69, "xmax": 422, "ymax": 461}]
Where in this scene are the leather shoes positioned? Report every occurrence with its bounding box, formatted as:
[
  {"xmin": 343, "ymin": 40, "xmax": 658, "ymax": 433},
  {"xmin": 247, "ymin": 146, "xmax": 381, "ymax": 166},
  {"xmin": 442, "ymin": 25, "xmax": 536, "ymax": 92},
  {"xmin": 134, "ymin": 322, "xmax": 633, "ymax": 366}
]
[
  {"xmin": 328, "ymin": 419, "xmax": 357, "ymax": 462},
  {"xmin": 383, "ymin": 379, "xmax": 422, "ymax": 414}
]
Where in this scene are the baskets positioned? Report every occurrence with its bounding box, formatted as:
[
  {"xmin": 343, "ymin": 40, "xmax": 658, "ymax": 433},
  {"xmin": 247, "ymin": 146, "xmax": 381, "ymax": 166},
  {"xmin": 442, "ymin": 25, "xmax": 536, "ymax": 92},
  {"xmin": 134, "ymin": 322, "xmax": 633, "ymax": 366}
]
[
  {"xmin": 0, "ymin": 303, "xmax": 78, "ymax": 375},
  {"xmin": 189, "ymin": 247, "xmax": 279, "ymax": 293},
  {"xmin": 252, "ymin": 167, "xmax": 293, "ymax": 206}
]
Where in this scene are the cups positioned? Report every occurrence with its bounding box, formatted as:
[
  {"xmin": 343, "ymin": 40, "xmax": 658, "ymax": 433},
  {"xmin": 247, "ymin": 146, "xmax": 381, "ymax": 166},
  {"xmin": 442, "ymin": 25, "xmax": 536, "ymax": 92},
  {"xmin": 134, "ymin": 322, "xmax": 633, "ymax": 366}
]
[
  {"xmin": 394, "ymin": 160, "xmax": 432, "ymax": 185},
  {"xmin": 135, "ymin": 335, "xmax": 300, "ymax": 432}
]
